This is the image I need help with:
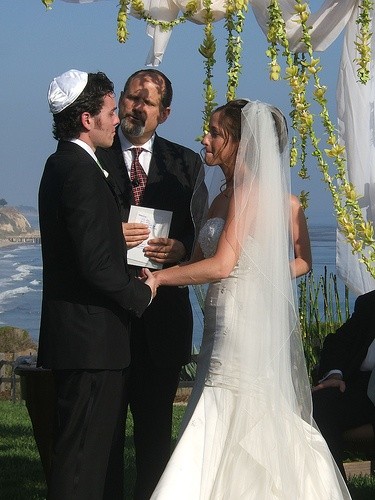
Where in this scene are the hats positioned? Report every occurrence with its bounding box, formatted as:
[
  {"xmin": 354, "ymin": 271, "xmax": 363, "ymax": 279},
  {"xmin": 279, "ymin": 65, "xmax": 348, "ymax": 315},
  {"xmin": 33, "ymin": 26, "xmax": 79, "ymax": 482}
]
[{"xmin": 47, "ymin": 70, "xmax": 88, "ymax": 114}]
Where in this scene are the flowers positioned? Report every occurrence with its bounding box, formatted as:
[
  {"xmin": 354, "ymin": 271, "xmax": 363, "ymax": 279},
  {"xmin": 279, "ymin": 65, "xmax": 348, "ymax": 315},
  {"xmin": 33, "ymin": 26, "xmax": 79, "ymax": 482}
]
[
  {"xmin": 41, "ymin": 0, "xmax": 54, "ymax": 10},
  {"xmin": 114, "ymin": 0, "xmax": 375, "ymax": 280}
]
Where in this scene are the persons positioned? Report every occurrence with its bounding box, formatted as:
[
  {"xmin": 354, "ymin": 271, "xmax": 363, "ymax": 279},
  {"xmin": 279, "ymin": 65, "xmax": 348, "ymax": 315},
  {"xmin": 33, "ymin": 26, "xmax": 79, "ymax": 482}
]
[
  {"xmin": 138, "ymin": 98, "xmax": 352, "ymax": 500},
  {"xmin": 35, "ymin": 68, "xmax": 159, "ymax": 500},
  {"xmin": 311, "ymin": 289, "xmax": 375, "ymax": 477},
  {"xmin": 94, "ymin": 67, "xmax": 209, "ymax": 500}
]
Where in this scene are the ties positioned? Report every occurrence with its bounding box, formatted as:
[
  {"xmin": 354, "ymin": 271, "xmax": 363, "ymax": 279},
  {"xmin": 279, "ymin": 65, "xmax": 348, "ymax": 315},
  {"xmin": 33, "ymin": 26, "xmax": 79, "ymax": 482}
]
[{"xmin": 130, "ymin": 147, "xmax": 148, "ymax": 206}]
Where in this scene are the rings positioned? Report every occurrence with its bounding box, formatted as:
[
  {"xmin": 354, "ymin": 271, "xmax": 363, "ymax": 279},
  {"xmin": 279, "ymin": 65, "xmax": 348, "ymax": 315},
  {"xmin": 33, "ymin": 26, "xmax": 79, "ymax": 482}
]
[{"xmin": 165, "ymin": 253, "xmax": 167, "ymax": 259}]
